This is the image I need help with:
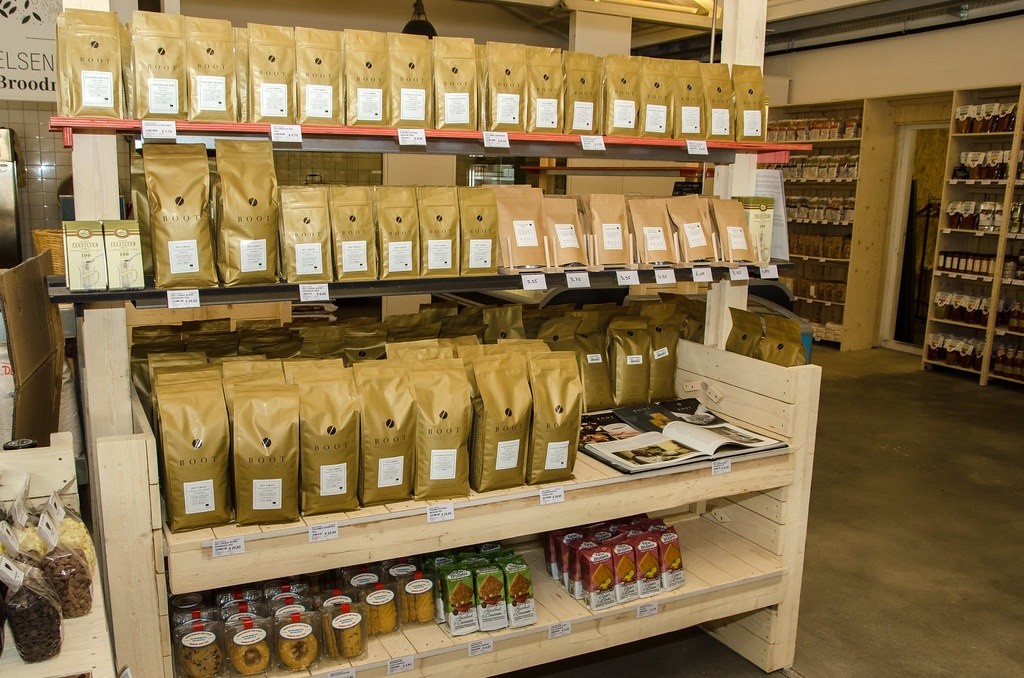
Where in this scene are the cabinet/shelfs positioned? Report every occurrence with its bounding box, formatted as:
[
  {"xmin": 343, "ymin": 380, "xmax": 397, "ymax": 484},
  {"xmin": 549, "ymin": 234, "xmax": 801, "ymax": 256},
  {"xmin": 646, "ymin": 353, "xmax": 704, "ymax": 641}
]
[{"xmin": 0, "ymin": 0, "xmax": 1024, "ymax": 678}]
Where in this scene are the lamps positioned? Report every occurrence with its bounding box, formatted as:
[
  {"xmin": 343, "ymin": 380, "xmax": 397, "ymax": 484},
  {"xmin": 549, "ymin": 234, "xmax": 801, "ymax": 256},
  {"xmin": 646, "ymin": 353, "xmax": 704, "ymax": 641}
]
[{"xmin": 402, "ymin": 0, "xmax": 438, "ymax": 39}]
